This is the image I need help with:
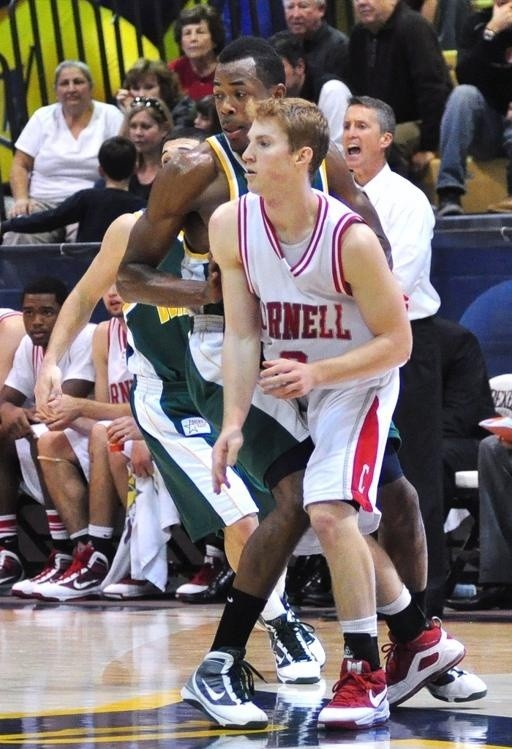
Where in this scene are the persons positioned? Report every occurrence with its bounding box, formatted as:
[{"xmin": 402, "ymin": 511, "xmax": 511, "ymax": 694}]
[
  {"xmin": 0, "ymin": 0, "xmax": 512, "ymax": 614},
  {"xmin": 182, "ymin": 98, "xmax": 487, "ymax": 729}
]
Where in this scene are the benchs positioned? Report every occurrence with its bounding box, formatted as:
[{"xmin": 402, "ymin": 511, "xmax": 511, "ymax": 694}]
[{"xmin": 411, "ymin": 50, "xmax": 512, "ymax": 216}]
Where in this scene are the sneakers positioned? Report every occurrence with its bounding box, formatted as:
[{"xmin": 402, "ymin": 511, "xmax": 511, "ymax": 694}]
[
  {"xmin": 173, "ymin": 543, "xmax": 341, "ymax": 610},
  {"xmin": 276, "ymin": 591, "xmax": 329, "ymax": 669},
  {"xmin": 437, "ymin": 190, "xmax": 463, "ymax": 217},
  {"xmin": 379, "ymin": 615, "xmax": 468, "ymax": 714},
  {"xmin": 104, "ymin": 552, "xmax": 183, "ymax": 604},
  {"xmin": 178, "ymin": 649, "xmax": 269, "ymax": 732},
  {"xmin": 11, "ymin": 546, "xmax": 73, "ymax": 601},
  {"xmin": 31, "ymin": 542, "xmax": 112, "ymax": 599},
  {"xmin": 312, "ymin": 653, "xmax": 392, "ymax": 733},
  {"xmin": 416, "ymin": 654, "xmax": 487, "ymax": 702},
  {"xmin": 259, "ymin": 615, "xmax": 322, "ymax": 687},
  {"xmin": 0, "ymin": 546, "xmax": 30, "ymax": 590},
  {"xmin": 485, "ymin": 198, "xmax": 511, "ymax": 214}
]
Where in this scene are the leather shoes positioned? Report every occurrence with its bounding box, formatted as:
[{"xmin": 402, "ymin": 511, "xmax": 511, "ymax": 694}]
[{"xmin": 439, "ymin": 582, "xmax": 511, "ymax": 613}]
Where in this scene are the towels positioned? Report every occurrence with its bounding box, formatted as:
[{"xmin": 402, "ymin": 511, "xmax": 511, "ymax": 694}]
[{"xmin": 98, "ymin": 460, "xmax": 182, "ymax": 592}]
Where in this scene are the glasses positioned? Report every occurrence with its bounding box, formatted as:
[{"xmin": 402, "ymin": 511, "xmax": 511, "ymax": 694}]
[{"xmin": 130, "ymin": 95, "xmax": 167, "ymax": 120}]
